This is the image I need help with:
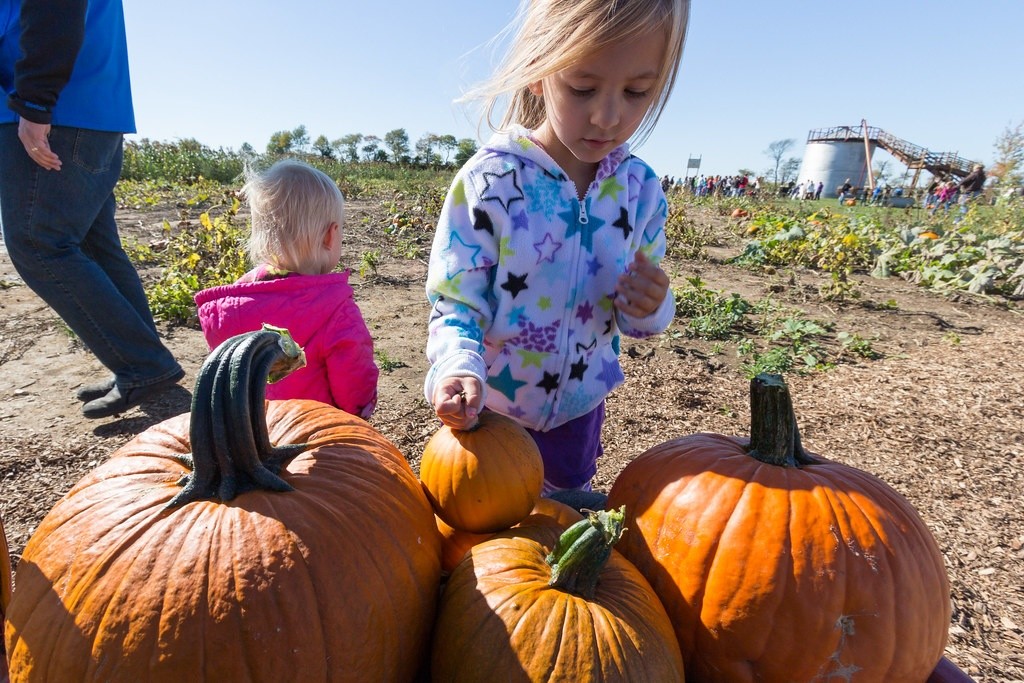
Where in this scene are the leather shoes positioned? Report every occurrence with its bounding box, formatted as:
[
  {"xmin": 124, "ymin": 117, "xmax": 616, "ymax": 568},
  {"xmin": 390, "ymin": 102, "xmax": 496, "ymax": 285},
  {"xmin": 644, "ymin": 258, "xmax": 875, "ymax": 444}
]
[{"xmin": 77, "ymin": 369, "xmax": 185, "ymax": 419}]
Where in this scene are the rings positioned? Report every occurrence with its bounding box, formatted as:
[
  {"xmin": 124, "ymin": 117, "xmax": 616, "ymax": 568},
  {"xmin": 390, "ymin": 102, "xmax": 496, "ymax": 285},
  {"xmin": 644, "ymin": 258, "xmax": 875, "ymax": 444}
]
[{"xmin": 32, "ymin": 148, "xmax": 38, "ymax": 151}]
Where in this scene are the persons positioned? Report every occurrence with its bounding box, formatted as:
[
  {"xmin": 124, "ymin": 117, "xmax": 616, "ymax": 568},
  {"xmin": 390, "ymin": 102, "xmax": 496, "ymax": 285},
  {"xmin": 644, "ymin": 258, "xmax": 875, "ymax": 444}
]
[
  {"xmin": 860, "ymin": 177, "xmax": 945, "ymax": 208},
  {"xmin": 788, "ymin": 179, "xmax": 809, "ymax": 201},
  {"xmin": 426, "ymin": 0, "xmax": 691, "ymax": 498},
  {"xmin": 197, "ymin": 160, "xmax": 378, "ymax": 418},
  {"xmin": 952, "ymin": 163, "xmax": 985, "ymax": 224},
  {"xmin": 838, "ymin": 178, "xmax": 852, "ymax": 206},
  {"xmin": 806, "ymin": 181, "xmax": 815, "ymax": 199},
  {"xmin": 0, "ymin": 0, "xmax": 186, "ymax": 417},
  {"xmin": 659, "ymin": 173, "xmax": 752, "ymax": 197},
  {"xmin": 932, "ymin": 181, "xmax": 957, "ymax": 216},
  {"xmin": 814, "ymin": 182, "xmax": 824, "ymax": 200}
]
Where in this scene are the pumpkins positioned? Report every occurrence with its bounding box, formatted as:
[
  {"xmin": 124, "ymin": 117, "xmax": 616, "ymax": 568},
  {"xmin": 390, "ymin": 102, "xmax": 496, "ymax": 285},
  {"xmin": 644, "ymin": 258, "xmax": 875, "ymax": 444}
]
[
  {"xmin": 435, "ymin": 497, "xmax": 588, "ymax": 592},
  {"xmin": 415, "ymin": 503, "xmax": 686, "ymax": 683},
  {"xmin": 420, "ymin": 394, "xmax": 545, "ymax": 535},
  {"xmin": 0, "ymin": 322, "xmax": 437, "ymax": 683},
  {"xmin": 596, "ymin": 373, "xmax": 951, "ymax": 683}
]
